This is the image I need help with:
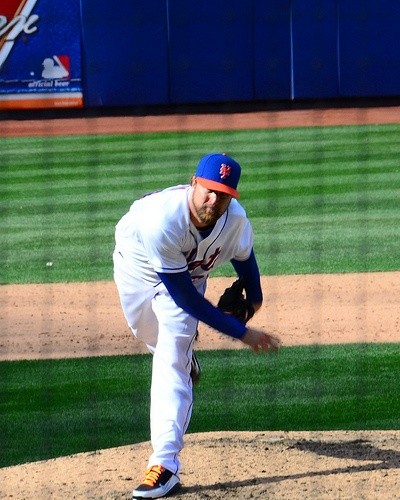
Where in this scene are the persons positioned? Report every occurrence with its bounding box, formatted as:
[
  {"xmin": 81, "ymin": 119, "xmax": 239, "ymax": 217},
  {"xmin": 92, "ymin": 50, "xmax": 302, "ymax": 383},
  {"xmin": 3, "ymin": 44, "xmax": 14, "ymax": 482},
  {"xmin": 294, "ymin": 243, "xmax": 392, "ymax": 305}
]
[{"xmin": 111, "ymin": 152, "xmax": 281, "ymax": 500}]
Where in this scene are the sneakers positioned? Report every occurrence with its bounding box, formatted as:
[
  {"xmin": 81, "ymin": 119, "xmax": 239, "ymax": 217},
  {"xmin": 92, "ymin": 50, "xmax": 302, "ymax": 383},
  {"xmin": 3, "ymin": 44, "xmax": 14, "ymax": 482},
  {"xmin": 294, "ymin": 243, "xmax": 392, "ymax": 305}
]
[
  {"xmin": 133, "ymin": 465, "xmax": 183, "ymax": 498},
  {"xmin": 190, "ymin": 350, "xmax": 201, "ymax": 381}
]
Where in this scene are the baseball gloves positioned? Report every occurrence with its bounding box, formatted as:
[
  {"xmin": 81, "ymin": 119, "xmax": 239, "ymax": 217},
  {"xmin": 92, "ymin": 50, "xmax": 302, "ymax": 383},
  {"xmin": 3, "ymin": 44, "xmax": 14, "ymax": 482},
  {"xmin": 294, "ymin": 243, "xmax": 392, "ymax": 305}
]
[{"xmin": 213, "ymin": 277, "xmax": 256, "ymax": 328}]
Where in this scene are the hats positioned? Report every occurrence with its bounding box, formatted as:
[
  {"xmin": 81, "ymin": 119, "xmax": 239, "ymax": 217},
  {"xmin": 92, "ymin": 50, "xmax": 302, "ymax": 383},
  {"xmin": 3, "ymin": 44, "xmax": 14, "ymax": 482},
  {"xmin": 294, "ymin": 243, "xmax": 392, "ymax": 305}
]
[{"xmin": 194, "ymin": 153, "xmax": 242, "ymax": 197}]
